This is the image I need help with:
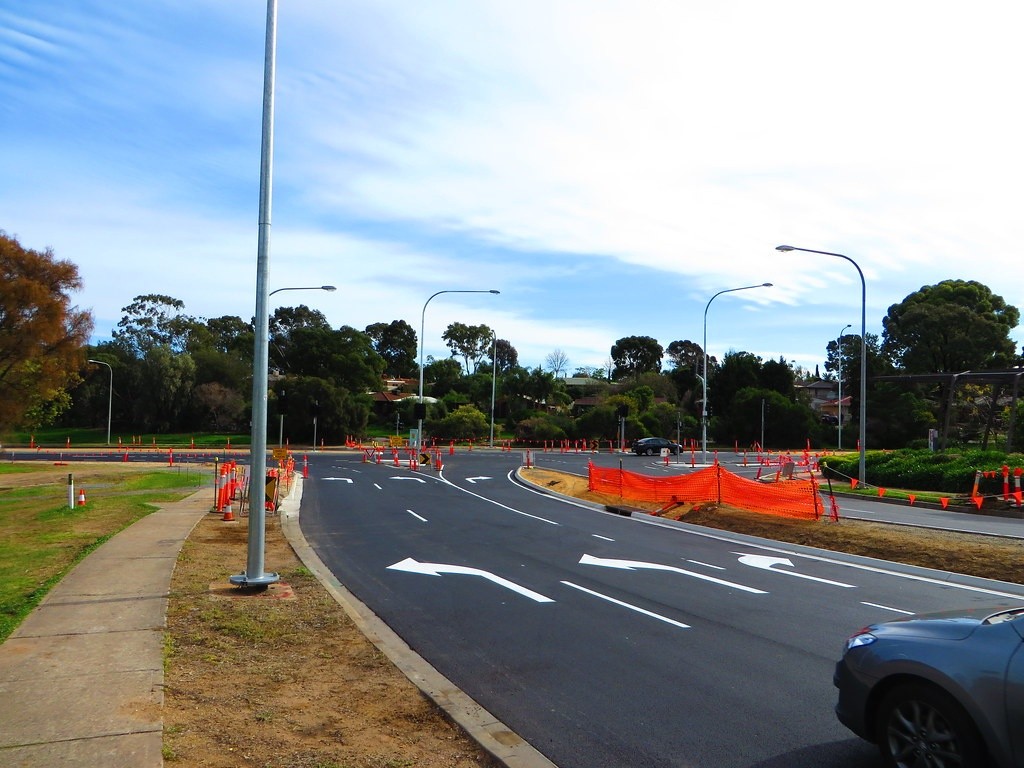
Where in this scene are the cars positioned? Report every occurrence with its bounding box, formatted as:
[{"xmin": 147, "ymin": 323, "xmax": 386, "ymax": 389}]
[
  {"xmin": 833, "ymin": 603, "xmax": 1024, "ymax": 768},
  {"xmin": 631, "ymin": 437, "xmax": 683, "ymax": 456}
]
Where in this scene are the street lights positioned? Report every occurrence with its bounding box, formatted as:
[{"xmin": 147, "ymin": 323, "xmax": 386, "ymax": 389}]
[
  {"xmin": 838, "ymin": 324, "xmax": 854, "ymax": 451},
  {"xmin": 418, "ymin": 289, "xmax": 500, "ymax": 456},
  {"xmin": 87, "ymin": 358, "xmax": 112, "ymax": 445},
  {"xmin": 268, "ymin": 281, "xmax": 336, "ymax": 304},
  {"xmin": 776, "ymin": 244, "xmax": 867, "ymax": 489},
  {"xmin": 701, "ymin": 282, "xmax": 772, "ymax": 461}
]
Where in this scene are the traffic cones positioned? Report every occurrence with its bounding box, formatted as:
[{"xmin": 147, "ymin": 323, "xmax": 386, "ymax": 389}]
[
  {"xmin": 221, "ymin": 499, "xmax": 234, "ymax": 521},
  {"xmin": 78, "ymin": 489, "xmax": 86, "ymax": 506}
]
